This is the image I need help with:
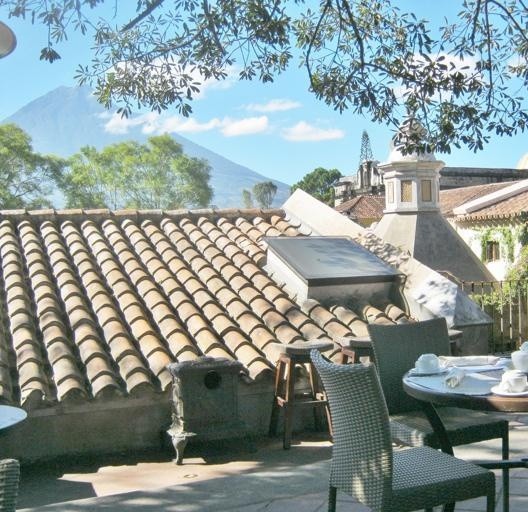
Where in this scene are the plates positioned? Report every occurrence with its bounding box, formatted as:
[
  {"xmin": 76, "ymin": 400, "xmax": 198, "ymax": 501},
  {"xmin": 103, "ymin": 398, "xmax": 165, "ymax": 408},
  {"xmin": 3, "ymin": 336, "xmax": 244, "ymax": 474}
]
[
  {"xmin": 409, "ymin": 367, "xmax": 447, "ymax": 374},
  {"xmin": 490, "ymin": 383, "xmax": 527, "ymax": 397}
]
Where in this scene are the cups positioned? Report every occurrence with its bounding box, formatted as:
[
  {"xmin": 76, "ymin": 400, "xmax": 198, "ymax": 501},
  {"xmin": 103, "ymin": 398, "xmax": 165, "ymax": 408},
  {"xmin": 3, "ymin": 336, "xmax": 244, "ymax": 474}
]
[
  {"xmin": 414, "ymin": 353, "xmax": 440, "ymax": 372},
  {"xmin": 511, "ymin": 351, "xmax": 528, "ymax": 370},
  {"xmin": 498, "ymin": 370, "xmax": 526, "ymax": 393}
]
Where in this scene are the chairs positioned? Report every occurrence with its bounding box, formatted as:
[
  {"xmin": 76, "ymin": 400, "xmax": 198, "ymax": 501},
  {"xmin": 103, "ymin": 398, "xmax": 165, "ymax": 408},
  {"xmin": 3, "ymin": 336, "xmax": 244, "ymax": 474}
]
[
  {"xmin": 301, "ymin": 345, "xmax": 499, "ymax": 511},
  {"xmin": 364, "ymin": 316, "xmax": 515, "ymax": 512}
]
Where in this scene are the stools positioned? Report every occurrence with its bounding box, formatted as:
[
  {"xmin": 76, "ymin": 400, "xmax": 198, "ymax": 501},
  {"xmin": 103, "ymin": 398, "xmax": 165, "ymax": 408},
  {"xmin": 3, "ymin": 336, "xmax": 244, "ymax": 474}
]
[
  {"xmin": 260, "ymin": 337, "xmax": 336, "ymax": 451},
  {"xmin": 339, "ymin": 334, "xmax": 375, "ymax": 365}
]
